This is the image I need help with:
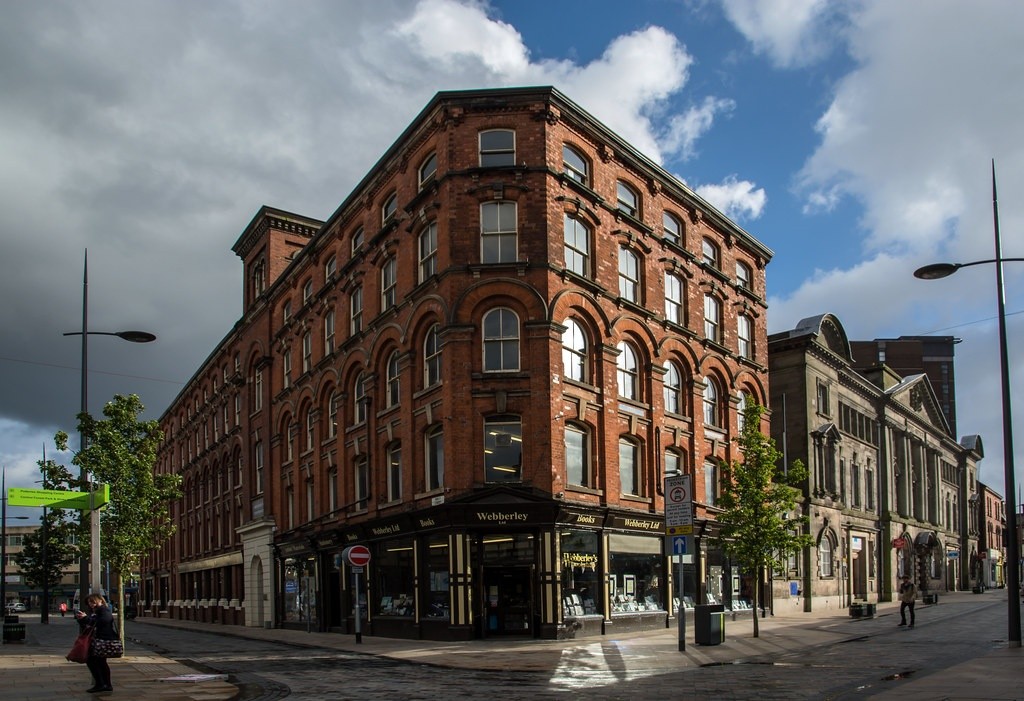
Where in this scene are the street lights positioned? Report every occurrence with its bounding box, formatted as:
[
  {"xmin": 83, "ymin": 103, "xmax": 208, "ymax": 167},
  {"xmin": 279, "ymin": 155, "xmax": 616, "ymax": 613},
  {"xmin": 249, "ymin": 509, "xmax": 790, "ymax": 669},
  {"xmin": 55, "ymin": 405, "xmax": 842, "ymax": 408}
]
[
  {"xmin": 0, "ymin": 464, "xmax": 31, "ymax": 615},
  {"xmin": 33, "ymin": 443, "xmax": 76, "ymax": 624},
  {"xmin": 913, "ymin": 157, "xmax": 1024, "ymax": 651},
  {"xmin": 62, "ymin": 247, "xmax": 158, "ymax": 633}
]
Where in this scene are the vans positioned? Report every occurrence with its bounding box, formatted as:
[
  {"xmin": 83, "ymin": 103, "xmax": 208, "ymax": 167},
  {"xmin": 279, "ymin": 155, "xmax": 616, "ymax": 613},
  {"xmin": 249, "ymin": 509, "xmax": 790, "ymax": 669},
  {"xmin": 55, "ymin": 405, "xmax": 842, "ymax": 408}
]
[{"xmin": 72, "ymin": 588, "xmax": 107, "ymax": 619}]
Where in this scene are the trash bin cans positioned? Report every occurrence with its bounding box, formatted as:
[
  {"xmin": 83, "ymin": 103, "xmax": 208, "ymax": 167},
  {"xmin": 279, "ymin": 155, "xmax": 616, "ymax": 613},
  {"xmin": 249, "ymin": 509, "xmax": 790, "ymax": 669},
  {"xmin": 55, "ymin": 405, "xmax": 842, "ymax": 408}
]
[{"xmin": 694, "ymin": 603, "xmax": 726, "ymax": 646}]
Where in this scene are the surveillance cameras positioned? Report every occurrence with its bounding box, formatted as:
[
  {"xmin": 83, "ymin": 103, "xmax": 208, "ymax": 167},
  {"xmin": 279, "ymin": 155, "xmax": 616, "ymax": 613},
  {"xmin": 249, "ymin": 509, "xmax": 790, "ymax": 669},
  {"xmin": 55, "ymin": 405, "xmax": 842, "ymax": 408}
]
[{"xmin": 559, "ymin": 492, "xmax": 564, "ymax": 497}]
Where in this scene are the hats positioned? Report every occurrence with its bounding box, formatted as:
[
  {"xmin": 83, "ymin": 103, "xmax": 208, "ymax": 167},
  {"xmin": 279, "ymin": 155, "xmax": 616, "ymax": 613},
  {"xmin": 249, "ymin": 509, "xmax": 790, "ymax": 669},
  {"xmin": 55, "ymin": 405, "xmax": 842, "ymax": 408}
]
[{"xmin": 902, "ymin": 575, "xmax": 910, "ymax": 579}]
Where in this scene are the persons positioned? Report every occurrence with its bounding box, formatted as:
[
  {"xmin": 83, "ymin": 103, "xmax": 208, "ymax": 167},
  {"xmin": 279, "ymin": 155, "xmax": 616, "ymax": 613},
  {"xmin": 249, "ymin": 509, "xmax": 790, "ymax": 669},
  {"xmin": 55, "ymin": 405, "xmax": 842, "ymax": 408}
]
[
  {"xmin": 897, "ymin": 576, "xmax": 917, "ymax": 627},
  {"xmin": 58, "ymin": 594, "xmax": 117, "ymax": 694}
]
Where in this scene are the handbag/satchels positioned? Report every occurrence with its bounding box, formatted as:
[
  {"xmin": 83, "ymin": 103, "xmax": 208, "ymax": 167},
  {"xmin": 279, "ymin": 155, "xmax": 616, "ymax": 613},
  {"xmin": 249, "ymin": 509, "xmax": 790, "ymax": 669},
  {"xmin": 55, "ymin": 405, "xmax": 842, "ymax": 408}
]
[
  {"xmin": 92, "ymin": 639, "xmax": 123, "ymax": 658},
  {"xmin": 66, "ymin": 635, "xmax": 91, "ymax": 663}
]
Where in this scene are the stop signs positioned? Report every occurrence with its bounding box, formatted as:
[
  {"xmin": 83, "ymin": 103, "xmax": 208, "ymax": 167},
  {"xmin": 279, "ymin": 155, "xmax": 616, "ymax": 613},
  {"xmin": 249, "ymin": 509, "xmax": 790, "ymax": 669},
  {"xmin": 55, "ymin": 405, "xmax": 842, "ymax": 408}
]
[{"xmin": 348, "ymin": 545, "xmax": 371, "ymax": 567}]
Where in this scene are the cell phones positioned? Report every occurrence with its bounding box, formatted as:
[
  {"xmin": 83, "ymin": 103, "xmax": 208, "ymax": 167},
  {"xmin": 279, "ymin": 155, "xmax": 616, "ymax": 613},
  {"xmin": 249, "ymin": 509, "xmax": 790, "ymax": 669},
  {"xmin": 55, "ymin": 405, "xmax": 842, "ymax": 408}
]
[{"xmin": 78, "ymin": 611, "xmax": 82, "ymax": 614}]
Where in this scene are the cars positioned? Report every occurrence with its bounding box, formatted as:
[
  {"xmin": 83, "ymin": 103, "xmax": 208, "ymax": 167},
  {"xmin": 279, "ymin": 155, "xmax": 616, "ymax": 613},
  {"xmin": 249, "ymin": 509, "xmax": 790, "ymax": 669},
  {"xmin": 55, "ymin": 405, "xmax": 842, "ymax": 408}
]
[{"xmin": 5, "ymin": 602, "xmax": 26, "ymax": 613}]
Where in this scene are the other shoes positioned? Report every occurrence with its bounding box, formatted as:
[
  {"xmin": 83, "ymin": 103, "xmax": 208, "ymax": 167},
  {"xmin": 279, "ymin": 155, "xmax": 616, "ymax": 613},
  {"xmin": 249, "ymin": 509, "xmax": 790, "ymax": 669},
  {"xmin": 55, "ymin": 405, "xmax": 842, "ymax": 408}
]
[
  {"xmin": 87, "ymin": 683, "xmax": 113, "ymax": 694},
  {"xmin": 908, "ymin": 625, "xmax": 914, "ymax": 628},
  {"xmin": 898, "ymin": 623, "xmax": 907, "ymax": 626}
]
